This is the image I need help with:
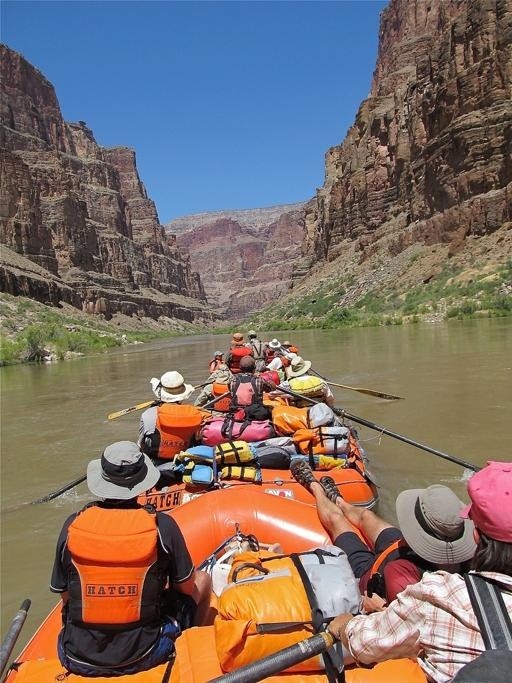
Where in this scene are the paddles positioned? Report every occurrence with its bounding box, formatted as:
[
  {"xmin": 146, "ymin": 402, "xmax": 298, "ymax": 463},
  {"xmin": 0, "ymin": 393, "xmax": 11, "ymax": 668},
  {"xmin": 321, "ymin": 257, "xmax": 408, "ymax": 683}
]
[
  {"xmin": 326, "ymin": 382, "xmax": 406, "ymax": 400},
  {"xmin": 109, "ymin": 381, "xmax": 213, "ymax": 421}
]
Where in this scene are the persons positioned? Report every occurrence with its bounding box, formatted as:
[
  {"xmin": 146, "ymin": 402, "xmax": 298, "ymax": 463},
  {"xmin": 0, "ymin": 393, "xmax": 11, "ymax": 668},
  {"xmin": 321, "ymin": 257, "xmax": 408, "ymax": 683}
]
[
  {"xmin": 138, "ymin": 328, "xmax": 333, "ymax": 464},
  {"xmin": 287, "ymin": 456, "xmax": 476, "ymax": 612},
  {"xmin": 50, "ymin": 441, "xmax": 212, "ymax": 671},
  {"xmin": 327, "ymin": 454, "xmax": 510, "ymax": 683}
]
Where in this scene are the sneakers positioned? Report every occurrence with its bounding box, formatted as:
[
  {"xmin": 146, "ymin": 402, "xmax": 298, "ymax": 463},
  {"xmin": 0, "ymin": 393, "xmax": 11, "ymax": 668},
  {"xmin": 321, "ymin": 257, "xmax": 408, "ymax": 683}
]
[
  {"xmin": 290, "ymin": 457, "xmax": 326, "ymax": 495},
  {"xmin": 319, "ymin": 475, "xmax": 343, "ymax": 503}
]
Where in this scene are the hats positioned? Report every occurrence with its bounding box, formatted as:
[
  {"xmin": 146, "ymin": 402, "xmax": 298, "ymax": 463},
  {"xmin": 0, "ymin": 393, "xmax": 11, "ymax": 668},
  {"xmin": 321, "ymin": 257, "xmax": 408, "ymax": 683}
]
[
  {"xmin": 268, "ymin": 339, "xmax": 292, "ymax": 349},
  {"xmin": 214, "ymin": 330, "xmax": 257, "ymax": 372},
  {"xmin": 395, "ymin": 484, "xmax": 478, "ymax": 564},
  {"xmin": 150, "ymin": 370, "xmax": 194, "ymax": 402},
  {"xmin": 287, "ymin": 357, "xmax": 311, "ymax": 377},
  {"xmin": 459, "ymin": 459, "xmax": 512, "ymax": 543},
  {"xmin": 86, "ymin": 440, "xmax": 160, "ymax": 500}
]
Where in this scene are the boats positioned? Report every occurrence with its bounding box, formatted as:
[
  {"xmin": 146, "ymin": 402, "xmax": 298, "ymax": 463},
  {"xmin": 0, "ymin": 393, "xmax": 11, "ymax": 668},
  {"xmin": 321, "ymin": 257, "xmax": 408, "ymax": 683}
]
[
  {"xmin": 137, "ymin": 392, "xmax": 381, "ymax": 516},
  {"xmin": 2, "ymin": 486, "xmax": 429, "ymax": 682},
  {"xmin": 194, "ymin": 347, "xmax": 326, "ymax": 412}
]
